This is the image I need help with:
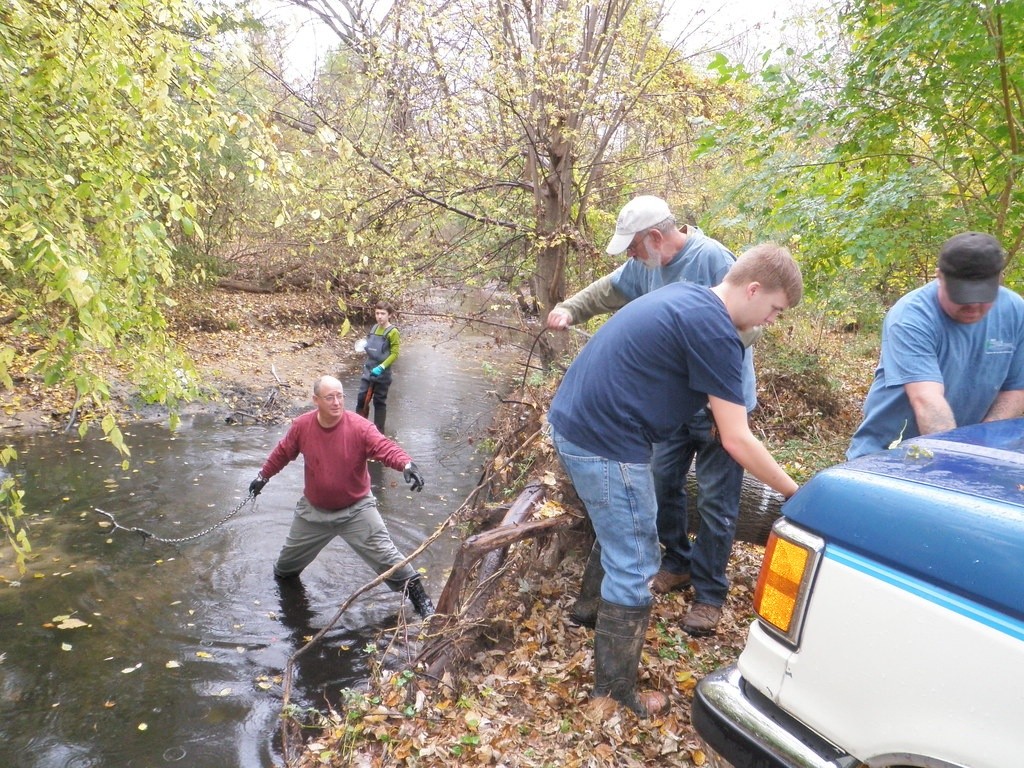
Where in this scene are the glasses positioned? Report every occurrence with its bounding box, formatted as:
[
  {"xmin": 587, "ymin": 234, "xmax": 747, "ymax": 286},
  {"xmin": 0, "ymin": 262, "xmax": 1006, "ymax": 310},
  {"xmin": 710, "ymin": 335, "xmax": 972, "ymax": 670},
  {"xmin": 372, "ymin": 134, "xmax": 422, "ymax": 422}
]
[
  {"xmin": 626, "ymin": 232, "xmax": 649, "ymax": 252},
  {"xmin": 316, "ymin": 394, "xmax": 347, "ymax": 401}
]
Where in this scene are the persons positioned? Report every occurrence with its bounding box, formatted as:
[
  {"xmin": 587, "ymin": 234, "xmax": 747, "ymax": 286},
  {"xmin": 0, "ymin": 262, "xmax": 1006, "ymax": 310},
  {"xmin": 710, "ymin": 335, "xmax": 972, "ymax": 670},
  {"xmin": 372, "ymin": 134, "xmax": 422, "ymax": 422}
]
[
  {"xmin": 250, "ymin": 375, "xmax": 436, "ymax": 619},
  {"xmin": 356, "ymin": 302, "xmax": 399, "ymax": 434},
  {"xmin": 846, "ymin": 231, "xmax": 1024, "ymax": 463},
  {"xmin": 546, "ymin": 241, "xmax": 804, "ymax": 713},
  {"xmin": 548, "ymin": 195, "xmax": 758, "ymax": 635}
]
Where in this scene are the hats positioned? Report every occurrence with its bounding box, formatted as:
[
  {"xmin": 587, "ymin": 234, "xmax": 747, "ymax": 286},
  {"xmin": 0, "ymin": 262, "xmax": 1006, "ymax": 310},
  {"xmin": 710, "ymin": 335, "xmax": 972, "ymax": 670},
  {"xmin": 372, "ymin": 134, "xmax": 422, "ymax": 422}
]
[
  {"xmin": 938, "ymin": 232, "xmax": 1005, "ymax": 305},
  {"xmin": 605, "ymin": 195, "xmax": 671, "ymax": 255}
]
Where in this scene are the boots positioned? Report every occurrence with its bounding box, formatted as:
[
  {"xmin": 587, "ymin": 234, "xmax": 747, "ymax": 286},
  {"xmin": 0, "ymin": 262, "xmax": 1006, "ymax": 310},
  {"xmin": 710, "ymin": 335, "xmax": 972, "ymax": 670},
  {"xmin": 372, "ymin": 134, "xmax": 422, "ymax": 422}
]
[
  {"xmin": 401, "ymin": 578, "xmax": 437, "ymax": 622},
  {"xmin": 569, "ymin": 536, "xmax": 606, "ymax": 627},
  {"xmin": 587, "ymin": 594, "xmax": 672, "ymax": 720}
]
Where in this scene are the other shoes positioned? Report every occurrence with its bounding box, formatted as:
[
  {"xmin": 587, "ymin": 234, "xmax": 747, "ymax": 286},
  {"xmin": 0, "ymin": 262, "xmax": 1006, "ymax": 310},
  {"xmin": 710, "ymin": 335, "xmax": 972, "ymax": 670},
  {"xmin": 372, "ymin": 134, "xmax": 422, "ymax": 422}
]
[
  {"xmin": 678, "ymin": 599, "xmax": 721, "ymax": 636},
  {"xmin": 652, "ymin": 566, "xmax": 692, "ymax": 594}
]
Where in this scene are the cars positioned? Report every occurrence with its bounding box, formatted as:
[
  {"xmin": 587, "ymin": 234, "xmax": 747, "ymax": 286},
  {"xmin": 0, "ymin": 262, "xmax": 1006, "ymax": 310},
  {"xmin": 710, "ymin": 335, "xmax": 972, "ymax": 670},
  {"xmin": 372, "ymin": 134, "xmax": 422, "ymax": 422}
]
[{"xmin": 690, "ymin": 417, "xmax": 1024, "ymax": 768}]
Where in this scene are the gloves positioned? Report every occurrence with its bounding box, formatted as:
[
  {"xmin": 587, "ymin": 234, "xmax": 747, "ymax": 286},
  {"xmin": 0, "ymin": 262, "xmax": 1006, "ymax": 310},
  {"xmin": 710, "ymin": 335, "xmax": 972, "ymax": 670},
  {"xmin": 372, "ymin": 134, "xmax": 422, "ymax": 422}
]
[
  {"xmin": 369, "ymin": 364, "xmax": 385, "ymax": 377},
  {"xmin": 402, "ymin": 460, "xmax": 424, "ymax": 492},
  {"xmin": 248, "ymin": 472, "xmax": 269, "ymax": 497}
]
